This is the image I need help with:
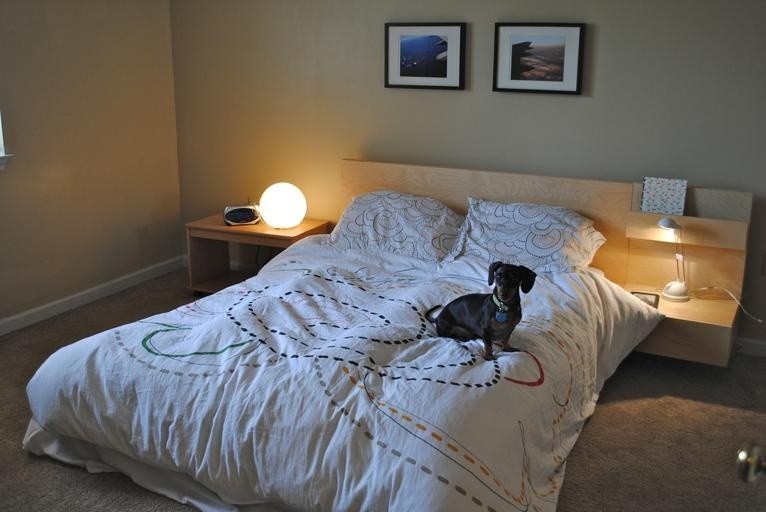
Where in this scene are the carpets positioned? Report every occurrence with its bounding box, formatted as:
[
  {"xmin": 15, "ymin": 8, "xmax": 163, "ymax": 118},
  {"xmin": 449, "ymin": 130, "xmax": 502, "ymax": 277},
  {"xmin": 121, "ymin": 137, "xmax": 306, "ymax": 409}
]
[{"xmin": 0, "ymin": 267, "xmax": 766, "ymax": 512}]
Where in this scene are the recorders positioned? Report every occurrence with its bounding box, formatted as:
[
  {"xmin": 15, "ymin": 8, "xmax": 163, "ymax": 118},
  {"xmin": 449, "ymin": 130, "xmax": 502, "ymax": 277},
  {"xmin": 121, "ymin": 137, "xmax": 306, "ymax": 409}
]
[{"xmin": 223, "ymin": 205, "xmax": 261, "ymax": 226}]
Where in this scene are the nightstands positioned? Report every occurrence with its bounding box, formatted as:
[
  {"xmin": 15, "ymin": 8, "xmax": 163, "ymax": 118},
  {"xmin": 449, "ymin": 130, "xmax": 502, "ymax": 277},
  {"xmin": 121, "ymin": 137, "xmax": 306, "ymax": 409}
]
[
  {"xmin": 624, "ymin": 182, "xmax": 755, "ymax": 368},
  {"xmin": 185, "ymin": 212, "xmax": 332, "ymax": 293}
]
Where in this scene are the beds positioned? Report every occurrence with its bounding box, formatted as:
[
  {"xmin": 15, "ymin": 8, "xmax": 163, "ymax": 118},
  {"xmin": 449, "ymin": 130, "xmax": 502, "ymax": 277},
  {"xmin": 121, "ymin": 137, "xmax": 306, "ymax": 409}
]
[{"xmin": 22, "ymin": 161, "xmax": 633, "ymax": 512}]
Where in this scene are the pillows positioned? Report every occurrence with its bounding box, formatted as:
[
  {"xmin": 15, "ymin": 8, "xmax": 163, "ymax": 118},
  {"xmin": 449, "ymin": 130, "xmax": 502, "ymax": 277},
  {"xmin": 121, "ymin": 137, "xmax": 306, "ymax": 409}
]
[
  {"xmin": 320, "ymin": 190, "xmax": 468, "ymax": 266},
  {"xmin": 461, "ymin": 193, "xmax": 607, "ymax": 275}
]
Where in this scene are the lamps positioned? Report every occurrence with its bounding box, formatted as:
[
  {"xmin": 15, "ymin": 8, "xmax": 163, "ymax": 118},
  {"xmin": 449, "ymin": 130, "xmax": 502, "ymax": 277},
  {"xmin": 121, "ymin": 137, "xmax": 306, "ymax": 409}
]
[
  {"xmin": 259, "ymin": 182, "xmax": 308, "ymax": 229},
  {"xmin": 658, "ymin": 218, "xmax": 689, "ymax": 303}
]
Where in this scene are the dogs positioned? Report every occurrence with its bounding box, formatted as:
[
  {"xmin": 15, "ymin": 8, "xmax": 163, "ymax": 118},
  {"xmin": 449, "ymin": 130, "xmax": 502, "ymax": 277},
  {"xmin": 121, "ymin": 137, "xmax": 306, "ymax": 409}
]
[{"xmin": 424, "ymin": 262, "xmax": 537, "ymax": 361}]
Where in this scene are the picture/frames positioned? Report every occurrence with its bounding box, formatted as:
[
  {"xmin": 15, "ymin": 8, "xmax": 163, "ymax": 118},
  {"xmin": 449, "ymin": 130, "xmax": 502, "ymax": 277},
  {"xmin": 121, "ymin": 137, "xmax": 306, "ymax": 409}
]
[
  {"xmin": 492, "ymin": 22, "xmax": 586, "ymax": 96},
  {"xmin": 631, "ymin": 292, "xmax": 659, "ymax": 308},
  {"xmin": 384, "ymin": 22, "xmax": 467, "ymax": 90}
]
